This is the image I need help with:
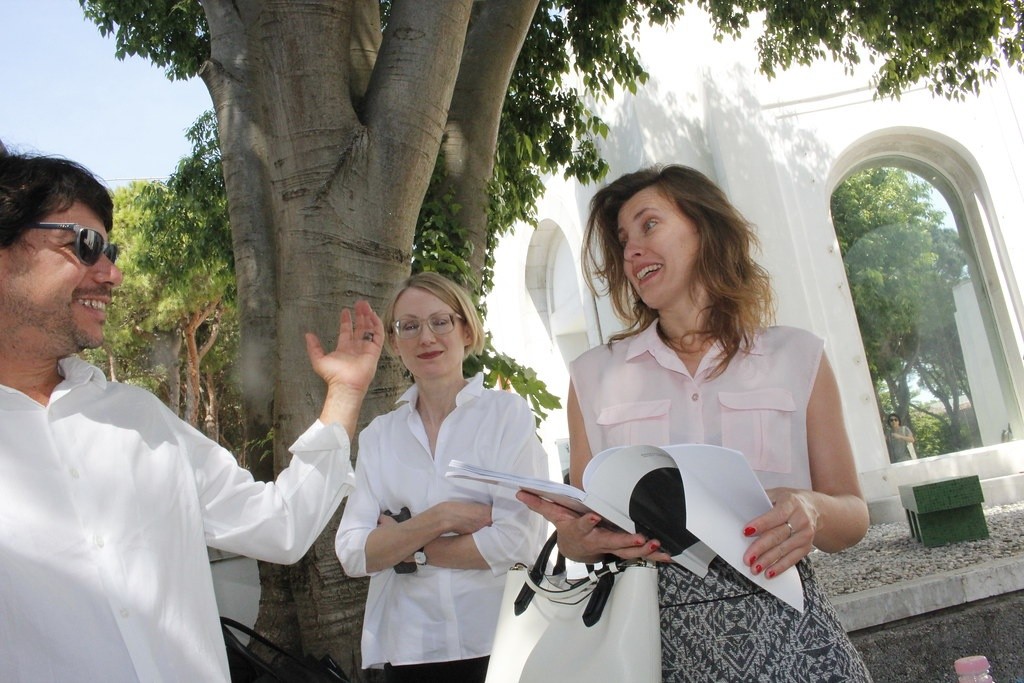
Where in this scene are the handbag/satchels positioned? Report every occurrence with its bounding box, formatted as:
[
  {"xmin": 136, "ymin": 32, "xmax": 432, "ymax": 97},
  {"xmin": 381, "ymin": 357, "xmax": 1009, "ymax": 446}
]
[
  {"xmin": 484, "ymin": 529, "xmax": 663, "ymax": 683},
  {"xmin": 220, "ymin": 617, "xmax": 350, "ymax": 683},
  {"xmin": 903, "ymin": 426, "xmax": 917, "ymax": 460}
]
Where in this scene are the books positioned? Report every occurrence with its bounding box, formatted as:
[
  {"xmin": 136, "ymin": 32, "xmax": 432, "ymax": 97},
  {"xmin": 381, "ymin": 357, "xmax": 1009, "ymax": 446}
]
[{"xmin": 444, "ymin": 444, "xmax": 804, "ymax": 614}]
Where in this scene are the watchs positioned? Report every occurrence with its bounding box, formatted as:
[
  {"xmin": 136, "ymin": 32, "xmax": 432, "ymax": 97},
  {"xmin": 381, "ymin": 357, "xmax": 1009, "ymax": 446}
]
[{"xmin": 414, "ymin": 547, "xmax": 427, "ymax": 565}]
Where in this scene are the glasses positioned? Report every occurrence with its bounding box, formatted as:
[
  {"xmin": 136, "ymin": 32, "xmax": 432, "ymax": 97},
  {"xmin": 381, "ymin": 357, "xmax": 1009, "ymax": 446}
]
[
  {"xmin": 23, "ymin": 222, "xmax": 120, "ymax": 265},
  {"xmin": 890, "ymin": 419, "xmax": 898, "ymax": 422},
  {"xmin": 391, "ymin": 313, "xmax": 467, "ymax": 339}
]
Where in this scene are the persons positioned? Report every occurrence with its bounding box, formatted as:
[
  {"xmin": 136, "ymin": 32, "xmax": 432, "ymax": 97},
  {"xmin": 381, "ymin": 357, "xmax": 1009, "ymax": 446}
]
[
  {"xmin": 884, "ymin": 414, "xmax": 914, "ymax": 464},
  {"xmin": 0, "ymin": 153, "xmax": 386, "ymax": 683},
  {"xmin": 515, "ymin": 164, "xmax": 870, "ymax": 683},
  {"xmin": 334, "ymin": 271, "xmax": 549, "ymax": 683}
]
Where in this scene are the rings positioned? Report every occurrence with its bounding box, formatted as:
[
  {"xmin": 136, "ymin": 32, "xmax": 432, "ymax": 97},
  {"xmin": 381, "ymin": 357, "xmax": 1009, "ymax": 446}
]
[
  {"xmin": 785, "ymin": 522, "xmax": 794, "ymax": 536},
  {"xmin": 364, "ymin": 332, "xmax": 373, "ymax": 341}
]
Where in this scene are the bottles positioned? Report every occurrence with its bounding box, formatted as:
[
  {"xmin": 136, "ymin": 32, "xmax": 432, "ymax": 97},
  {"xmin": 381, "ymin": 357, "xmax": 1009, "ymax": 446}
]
[{"xmin": 955, "ymin": 656, "xmax": 995, "ymax": 683}]
